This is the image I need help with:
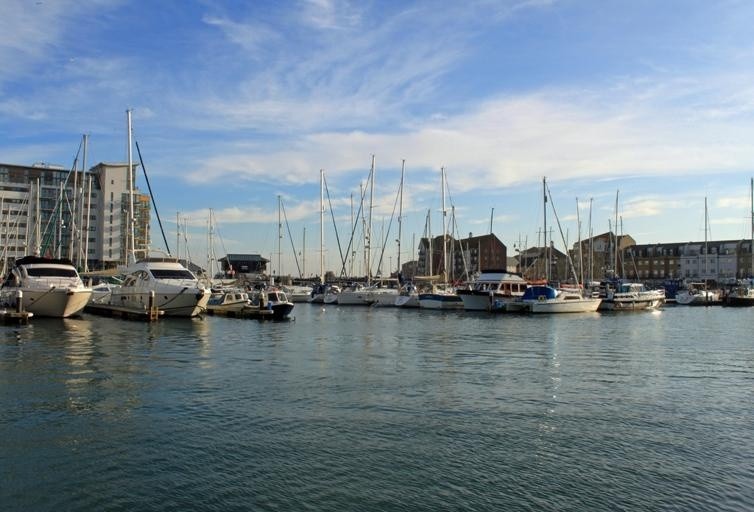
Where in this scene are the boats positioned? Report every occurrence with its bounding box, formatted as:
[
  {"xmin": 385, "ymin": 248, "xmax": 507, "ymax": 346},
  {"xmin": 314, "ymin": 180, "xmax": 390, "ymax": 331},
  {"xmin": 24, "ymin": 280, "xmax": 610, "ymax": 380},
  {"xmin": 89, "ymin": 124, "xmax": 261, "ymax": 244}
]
[{"xmin": 88, "ymin": 283, "xmax": 118, "ymax": 303}]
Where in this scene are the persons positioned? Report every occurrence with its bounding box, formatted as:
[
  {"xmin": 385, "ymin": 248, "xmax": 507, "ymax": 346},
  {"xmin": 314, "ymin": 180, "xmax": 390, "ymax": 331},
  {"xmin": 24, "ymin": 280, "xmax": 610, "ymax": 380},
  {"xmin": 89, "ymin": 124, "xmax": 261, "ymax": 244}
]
[{"xmin": 258, "ymin": 288, "xmax": 271, "ymax": 310}]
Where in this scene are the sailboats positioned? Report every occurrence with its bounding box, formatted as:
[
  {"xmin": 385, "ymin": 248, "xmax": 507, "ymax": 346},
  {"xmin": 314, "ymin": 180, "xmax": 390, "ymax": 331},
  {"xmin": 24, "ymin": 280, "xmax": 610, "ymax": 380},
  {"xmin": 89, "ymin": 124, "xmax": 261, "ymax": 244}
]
[
  {"xmin": 111, "ymin": 108, "xmax": 212, "ymax": 317},
  {"xmin": 0, "ymin": 175, "xmax": 92, "ymax": 320}
]
